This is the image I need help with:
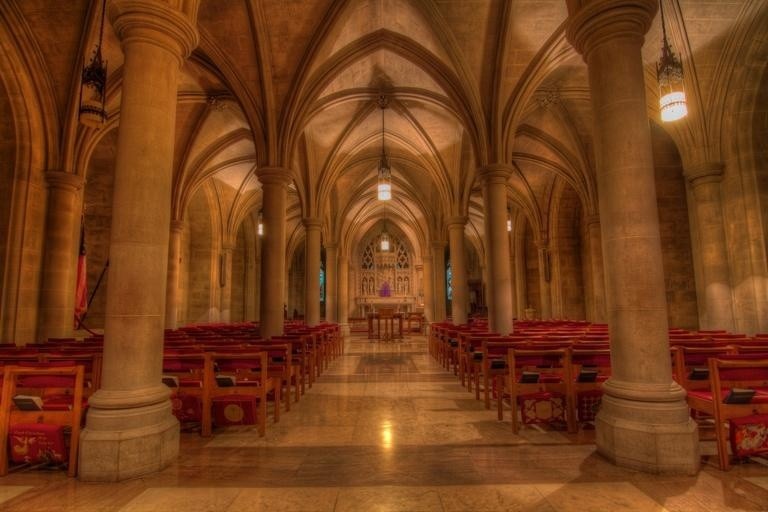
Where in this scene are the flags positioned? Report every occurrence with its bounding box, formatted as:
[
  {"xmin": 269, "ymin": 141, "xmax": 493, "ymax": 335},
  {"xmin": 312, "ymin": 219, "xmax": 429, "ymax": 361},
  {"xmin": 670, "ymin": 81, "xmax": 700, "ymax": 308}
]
[{"xmin": 72, "ymin": 214, "xmax": 89, "ymax": 330}]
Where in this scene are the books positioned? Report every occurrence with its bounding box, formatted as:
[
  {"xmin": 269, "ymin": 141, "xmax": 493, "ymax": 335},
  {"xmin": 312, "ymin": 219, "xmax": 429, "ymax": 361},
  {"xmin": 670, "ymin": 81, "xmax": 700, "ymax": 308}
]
[
  {"xmin": 577, "ymin": 370, "xmax": 597, "ymax": 382},
  {"xmin": 161, "ymin": 375, "xmax": 180, "ymax": 388},
  {"xmin": 11, "ymin": 394, "xmax": 44, "ymax": 412},
  {"xmin": 721, "ymin": 389, "xmax": 755, "ymax": 404},
  {"xmin": 214, "ymin": 375, "xmax": 236, "ymax": 388},
  {"xmin": 489, "ymin": 359, "xmax": 505, "ymax": 369},
  {"xmin": 472, "ymin": 351, "xmax": 483, "ymax": 360},
  {"xmin": 518, "ymin": 371, "xmax": 539, "ymax": 383},
  {"xmin": 684, "ymin": 366, "xmax": 710, "ymax": 380}
]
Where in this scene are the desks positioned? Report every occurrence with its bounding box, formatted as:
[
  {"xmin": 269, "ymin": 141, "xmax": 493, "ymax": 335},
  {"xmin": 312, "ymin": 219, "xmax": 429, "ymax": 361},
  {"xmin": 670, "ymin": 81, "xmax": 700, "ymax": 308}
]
[{"xmin": 366, "ymin": 311, "xmax": 404, "ymax": 342}]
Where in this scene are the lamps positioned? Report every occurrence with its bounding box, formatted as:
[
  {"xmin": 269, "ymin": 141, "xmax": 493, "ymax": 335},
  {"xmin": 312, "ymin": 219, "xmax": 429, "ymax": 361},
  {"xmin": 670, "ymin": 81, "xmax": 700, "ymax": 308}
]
[
  {"xmin": 650, "ymin": 1, "xmax": 691, "ymax": 126},
  {"xmin": 380, "ymin": 205, "xmax": 392, "ymax": 253},
  {"xmin": 374, "ymin": 90, "xmax": 395, "ymax": 204}
]
